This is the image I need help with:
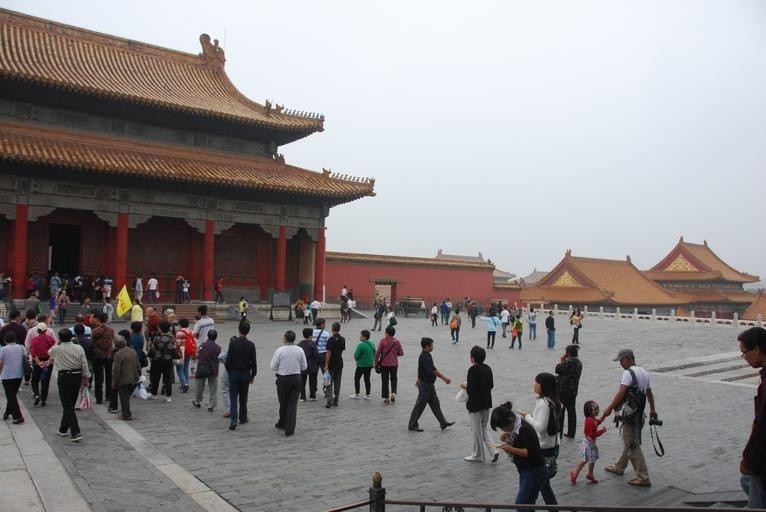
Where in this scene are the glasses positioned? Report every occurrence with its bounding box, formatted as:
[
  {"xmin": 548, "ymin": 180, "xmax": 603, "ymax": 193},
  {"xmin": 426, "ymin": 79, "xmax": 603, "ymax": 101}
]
[{"xmin": 741, "ymin": 353, "xmax": 745, "ymax": 357}]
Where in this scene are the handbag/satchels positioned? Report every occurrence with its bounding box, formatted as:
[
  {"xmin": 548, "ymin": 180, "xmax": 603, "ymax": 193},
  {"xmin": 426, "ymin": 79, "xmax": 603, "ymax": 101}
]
[
  {"xmin": 156, "ymin": 291, "xmax": 159, "ymax": 298},
  {"xmin": 23, "ymin": 355, "xmax": 32, "ymax": 381},
  {"xmin": 450, "ymin": 319, "xmax": 458, "ymax": 329},
  {"xmin": 390, "ymin": 317, "xmax": 397, "ymax": 326},
  {"xmin": 376, "ymin": 361, "xmax": 381, "ymax": 373},
  {"xmin": 173, "ymin": 346, "xmax": 185, "ymax": 365},
  {"xmin": 188, "ymin": 358, "xmax": 198, "ymax": 378},
  {"xmin": 546, "ymin": 456, "xmax": 558, "ymax": 478},
  {"xmin": 578, "ymin": 324, "xmax": 582, "ymax": 328},
  {"xmin": 512, "ymin": 329, "xmax": 518, "ymax": 337},
  {"xmin": 79, "ymin": 387, "xmax": 91, "ymax": 410}
]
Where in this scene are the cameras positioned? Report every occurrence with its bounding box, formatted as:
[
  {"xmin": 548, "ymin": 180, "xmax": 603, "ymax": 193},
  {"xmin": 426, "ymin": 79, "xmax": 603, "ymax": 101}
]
[{"xmin": 649, "ymin": 416, "xmax": 663, "ymax": 426}]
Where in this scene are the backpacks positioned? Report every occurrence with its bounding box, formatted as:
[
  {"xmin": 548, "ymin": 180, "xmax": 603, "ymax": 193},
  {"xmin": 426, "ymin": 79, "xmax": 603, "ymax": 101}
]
[
  {"xmin": 176, "ymin": 330, "xmax": 196, "ymax": 355},
  {"xmin": 614, "ymin": 368, "xmax": 646, "ymax": 423}
]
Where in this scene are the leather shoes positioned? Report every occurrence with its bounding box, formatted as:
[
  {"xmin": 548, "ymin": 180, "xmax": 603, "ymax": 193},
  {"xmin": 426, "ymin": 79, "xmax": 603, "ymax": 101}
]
[
  {"xmin": 409, "ymin": 428, "xmax": 424, "ymax": 432},
  {"xmin": 442, "ymin": 421, "xmax": 455, "ymax": 429}
]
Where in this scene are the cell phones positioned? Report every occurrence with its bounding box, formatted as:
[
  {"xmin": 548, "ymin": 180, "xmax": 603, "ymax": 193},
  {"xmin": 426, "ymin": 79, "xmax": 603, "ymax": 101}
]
[{"xmin": 517, "ymin": 409, "xmax": 525, "ymax": 414}]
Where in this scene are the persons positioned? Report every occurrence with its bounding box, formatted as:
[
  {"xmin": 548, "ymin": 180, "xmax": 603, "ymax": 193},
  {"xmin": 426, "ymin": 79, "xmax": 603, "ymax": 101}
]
[
  {"xmin": 461, "ymin": 346, "xmax": 499, "ymax": 462},
  {"xmin": 408, "ymin": 337, "xmax": 455, "ymax": 432},
  {"xmin": 569, "ymin": 400, "xmax": 607, "ymax": 485},
  {"xmin": 518, "ymin": 373, "xmax": 559, "ymax": 512},
  {"xmin": 737, "ymin": 327, "xmax": 766, "ymax": 509},
  {"xmin": 491, "ymin": 401, "xmax": 548, "ymax": 512},
  {"xmin": 556, "ymin": 344, "xmax": 583, "ymax": 438},
  {"xmin": 431, "ymin": 297, "xmax": 584, "ymax": 350},
  {"xmin": 0, "ymin": 268, "xmax": 404, "ymax": 442},
  {"xmin": 604, "ymin": 349, "xmax": 657, "ymax": 487}
]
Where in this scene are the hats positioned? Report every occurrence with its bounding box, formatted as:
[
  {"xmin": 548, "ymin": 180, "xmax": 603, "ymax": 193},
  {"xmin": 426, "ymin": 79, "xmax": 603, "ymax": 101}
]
[
  {"xmin": 613, "ymin": 349, "xmax": 632, "ymax": 361},
  {"xmin": 37, "ymin": 322, "xmax": 47, "ymax": 332}
]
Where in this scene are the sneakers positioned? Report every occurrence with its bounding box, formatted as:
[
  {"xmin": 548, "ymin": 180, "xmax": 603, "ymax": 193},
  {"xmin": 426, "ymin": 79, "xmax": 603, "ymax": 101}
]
[
  {"xmin": 605, "ymin": 466, "xmax": 624, "ymax": 475},
  {"xmin": 564, "ymin": 432, "xmax": 575, "ymax": 438},
  {"xmin": 464, "ymin": 455, "xmax": 483, "ymax": 462},
  {"xmin": 491, "ymin": 450, "xmax": 499, "ymax": 462},
  {"xmin": 33, "ymin": 395, "xmax": 47, "ymax": 406},
  {"xmin": 222, "ymin": 408, "xmax": 248, "ymax": 429},
  {"xmin": 145, "ymin": 383, "xmax": 189, "ymax": 402},
  {"xmin": 570, "ymin": 471, "xmax": 577, "ymax": 484},
  {"xmin": 629, "ymin": 479, "xmax": 650, "ymax": 487},
  {"xmin": 586, "ymin": 474, "xmax": 598, "ymax": 483},
  {"xmin": 300, "ymin": 397, "xmax": 338, "ymax": 407},
  {"xmin": 192, "ymin": 400, "xmax": 213, "ymax": 410},
  {"xmin": 384, "ymin": 395, "xmax": 395, "ymax": 403},
  {"xmin": 108, "ymin": 407, "xmax": 133, "ymax": 420},
  {"xmin": 349, "ymin": 395, "xmax": 370, "ymax": 400},
  {"xmin": 3, "ymin": 413, "xmax": 24, "ymax": 424},
  {"xmin": 275, "ymin": 423, "xmax": 295, "ymax": 436},
  {"xmin": 57, "ymin": 431, "xmax": 82, "ymax": 441}
]
